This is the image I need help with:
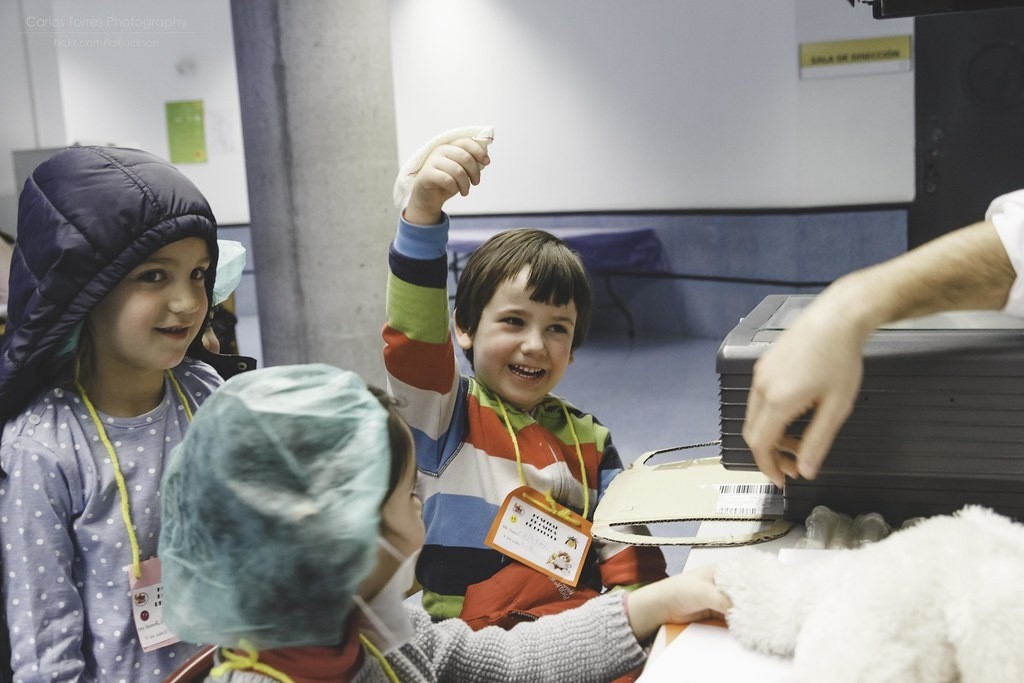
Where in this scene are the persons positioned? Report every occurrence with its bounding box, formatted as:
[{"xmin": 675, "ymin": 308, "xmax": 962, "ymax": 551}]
[
  {"xmin": 164, "ymin": 366, "xmax": 729, "ymax": 682},
  {"xmin": 202, "ymin": 240, "xmax": 247, "ymax": 354},
  {"xmin": 382, "ymin": 138, "xmax": 674, "ymax": 683},
  {"xmin": 739, "ymin": 186, "xmax": 1023, "ymax": 490},
  {"xmin": 1, "ymin": 145, "xmax": 225, "ymax": 681}
]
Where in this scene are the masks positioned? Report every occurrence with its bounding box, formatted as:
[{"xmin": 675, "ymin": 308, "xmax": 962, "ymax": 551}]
[{"xmin": 352, "ymin": 538, "xmax": 422, "ymax": 658}]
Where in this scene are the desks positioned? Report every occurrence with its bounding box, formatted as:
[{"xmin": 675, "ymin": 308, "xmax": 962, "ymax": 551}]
[
  {"xmin": 635, "ymin": 520, "xmax": 814, "ymax": 683},
  {"xmin": 444, "ymin": 226, "xmax": 663, "ymax": 348}
]
